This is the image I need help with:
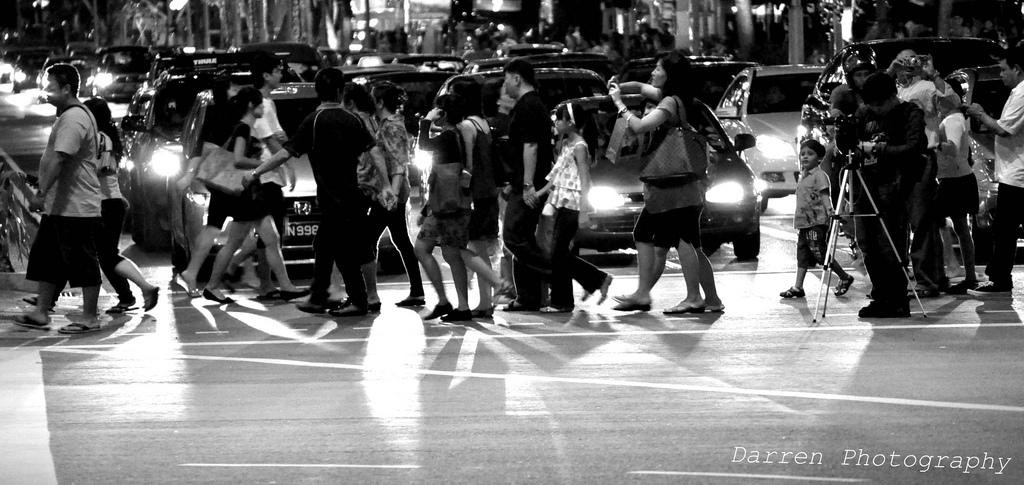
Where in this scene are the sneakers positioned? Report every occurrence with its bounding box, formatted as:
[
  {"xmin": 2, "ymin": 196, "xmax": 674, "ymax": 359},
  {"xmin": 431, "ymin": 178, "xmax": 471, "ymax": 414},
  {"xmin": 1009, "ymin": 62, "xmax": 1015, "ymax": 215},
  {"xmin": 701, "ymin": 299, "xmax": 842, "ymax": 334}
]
[
  {"xmin": 908, "ymin": 267, "xmax": 978, "ymax": 299},
  {"xmin": 967, "ymin": 281, "xmax": 1014, "ymax": 297},
  {"xmin": 858, "ymin": 301, "xmax": 912, "ymax": 318}
]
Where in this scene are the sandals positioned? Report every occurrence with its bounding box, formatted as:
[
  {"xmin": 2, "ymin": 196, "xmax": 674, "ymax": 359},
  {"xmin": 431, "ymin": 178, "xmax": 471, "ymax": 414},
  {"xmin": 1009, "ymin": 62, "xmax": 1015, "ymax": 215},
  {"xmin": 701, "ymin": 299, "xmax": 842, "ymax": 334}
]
[
  {"xmin": 834, "ymin": 274, "xmax": 855, "ymax": 296},
  {"xmin": 779, "ymin": 287, "xmax": 806, "ymax": 299}
]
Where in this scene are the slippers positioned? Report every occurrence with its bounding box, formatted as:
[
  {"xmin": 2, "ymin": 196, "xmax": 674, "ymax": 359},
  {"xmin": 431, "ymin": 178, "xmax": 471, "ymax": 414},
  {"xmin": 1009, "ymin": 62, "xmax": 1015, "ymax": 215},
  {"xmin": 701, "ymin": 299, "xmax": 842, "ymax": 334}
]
[
  {"xmin": 613, "ymin": 295, "xmax": 653, "ymax": 311},
  {"xmin": 105, "ymin": 304, "xmax": 140, "ymax": 314},
  {"xmin": 11, "ymin": 314, "xmax": 51, "ymax": 331},
  {"xmin": 502, "ymin": 298, "xmax": 538, "ymax": 312},
  {"xmin": 22, "ymin": 296, "xmax": 56, "ymax": 313},
  {"xmin": 58, "ymin": 322, "xmax": 102, "ymax": 335},
  {"xmin": 663, "ymin": 301, "xmax": 725, "ymax": 314},
  {"xmin": 144, "ymin": 287, "xmax": 160, "ymax": 312}
]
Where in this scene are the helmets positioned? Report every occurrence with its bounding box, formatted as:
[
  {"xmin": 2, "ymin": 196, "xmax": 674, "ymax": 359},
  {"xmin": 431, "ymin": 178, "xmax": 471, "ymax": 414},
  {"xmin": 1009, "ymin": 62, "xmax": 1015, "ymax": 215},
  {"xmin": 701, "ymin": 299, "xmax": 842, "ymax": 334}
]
[{"xmin": 843, "ymin": 50, "xmax": 878, "ymax": 85}]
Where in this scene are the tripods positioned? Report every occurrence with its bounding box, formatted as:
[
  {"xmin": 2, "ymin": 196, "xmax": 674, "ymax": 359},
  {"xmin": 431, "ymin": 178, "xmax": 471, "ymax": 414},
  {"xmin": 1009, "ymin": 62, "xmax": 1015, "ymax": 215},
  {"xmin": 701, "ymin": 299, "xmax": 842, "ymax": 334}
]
[{"xmin": 812, "ymin": 154, "xmax": 929, "ymax": 324}]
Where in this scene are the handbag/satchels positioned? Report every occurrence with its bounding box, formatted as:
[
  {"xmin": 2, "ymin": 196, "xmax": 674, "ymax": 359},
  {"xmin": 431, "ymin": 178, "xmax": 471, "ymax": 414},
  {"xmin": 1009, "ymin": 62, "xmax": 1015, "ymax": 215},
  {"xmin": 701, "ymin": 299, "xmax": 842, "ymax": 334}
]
[
  {"xmin": 489, "ymin": 139, "xmax": 517, "ymax": 189},
  {"xmin": 195, "ymin": 142, "xmax": 258, "ymax": 197},
  {"xmin": 637, "ymin": 127, "xmax": 707, "ymax": 187},
  {"xmin": 605, "ymin": 110, "xmax": 643, "ymax": 169},
  {"xmin": 428, "ymin": 160, "xmax": 475, "ymax": 217}
]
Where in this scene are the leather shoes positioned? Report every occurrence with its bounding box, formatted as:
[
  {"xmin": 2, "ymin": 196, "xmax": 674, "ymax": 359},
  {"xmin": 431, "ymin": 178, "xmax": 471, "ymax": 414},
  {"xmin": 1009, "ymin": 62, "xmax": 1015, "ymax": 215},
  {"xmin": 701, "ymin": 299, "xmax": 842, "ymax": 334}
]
[
  {"xmin": 538, "ymin": 306, "xmax": 575, "ymax": 313},
  {"xmin": 471, "ymin": 309, "xmax": 495, "ymax": 317},
  {"xmin": 346, "ymin": 297, "xmax": 382, "ymax": 312},
  {"xmin": 203, "ymin": 287, "xmax": 235, "ymax": 304},
  {"xmin": 327, "ymin": 306, "xmax": 369, "ymax": 317},
  {"xmin": 423, "ymin": 302, "xmax": 453, "ymax": 321},
  {"xmin": 257, "ymin": 289, "xmax": 280, "ymax": 300},
  {"xmin": 395, "ymin": 298, "xmax": 427, "ymax": 307},
  {"xmin": 295, "ymin": 304, "xmax": 327, "ymax": 314},
  {"xmin": 440, "ymin": 308, "xmax": 472, "ymax": 323},
  {"xmin": 281, "ymin": 287, "xmax": 311, "ymax": 303},
  {"xmin": 598, "ymin": 273, "xmax": 612, "ymax": 305}
]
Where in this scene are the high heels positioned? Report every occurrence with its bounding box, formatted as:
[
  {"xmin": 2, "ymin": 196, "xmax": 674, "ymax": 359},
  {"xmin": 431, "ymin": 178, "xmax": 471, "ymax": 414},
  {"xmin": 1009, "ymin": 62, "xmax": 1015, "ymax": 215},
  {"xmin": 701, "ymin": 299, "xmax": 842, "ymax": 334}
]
[{"xmin": 175, "ymin": 273, "xmax": 201, "ymax": 298}]
